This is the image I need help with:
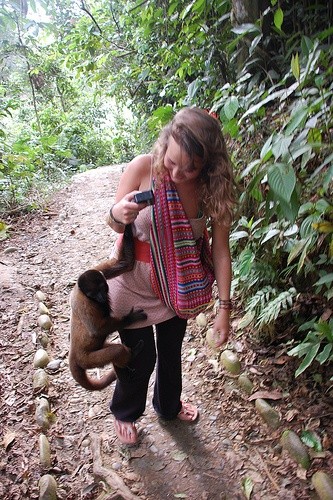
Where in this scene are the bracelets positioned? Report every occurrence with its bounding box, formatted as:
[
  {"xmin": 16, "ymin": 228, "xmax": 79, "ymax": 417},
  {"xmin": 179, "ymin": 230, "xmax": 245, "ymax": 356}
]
[
  {"xmin": 109, "ymin": 205, "xmax": 122, "ymax": 223},
  {"xmin": 219, "ymin": 298, "xmax": 232, "ymax": 311}
]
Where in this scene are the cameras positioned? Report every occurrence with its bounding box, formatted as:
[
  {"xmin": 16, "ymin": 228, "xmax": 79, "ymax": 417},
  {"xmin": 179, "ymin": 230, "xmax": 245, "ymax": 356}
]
[{"xmin": 134, "ymin": 190, "xmax": 155, "ymax": 206}]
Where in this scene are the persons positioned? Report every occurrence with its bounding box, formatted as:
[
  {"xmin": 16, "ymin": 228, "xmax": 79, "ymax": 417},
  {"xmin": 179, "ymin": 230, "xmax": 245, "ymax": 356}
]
[{"xmin": 105, "ymin": 106, "xmax": 235, "ymax": 446}]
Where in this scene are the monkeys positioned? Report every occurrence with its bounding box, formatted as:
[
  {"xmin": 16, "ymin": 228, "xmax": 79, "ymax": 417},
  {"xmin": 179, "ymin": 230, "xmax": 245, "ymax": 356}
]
[{"xmin": 69, "ymin": 222, "xmax": 148, "ymax": 392}]
